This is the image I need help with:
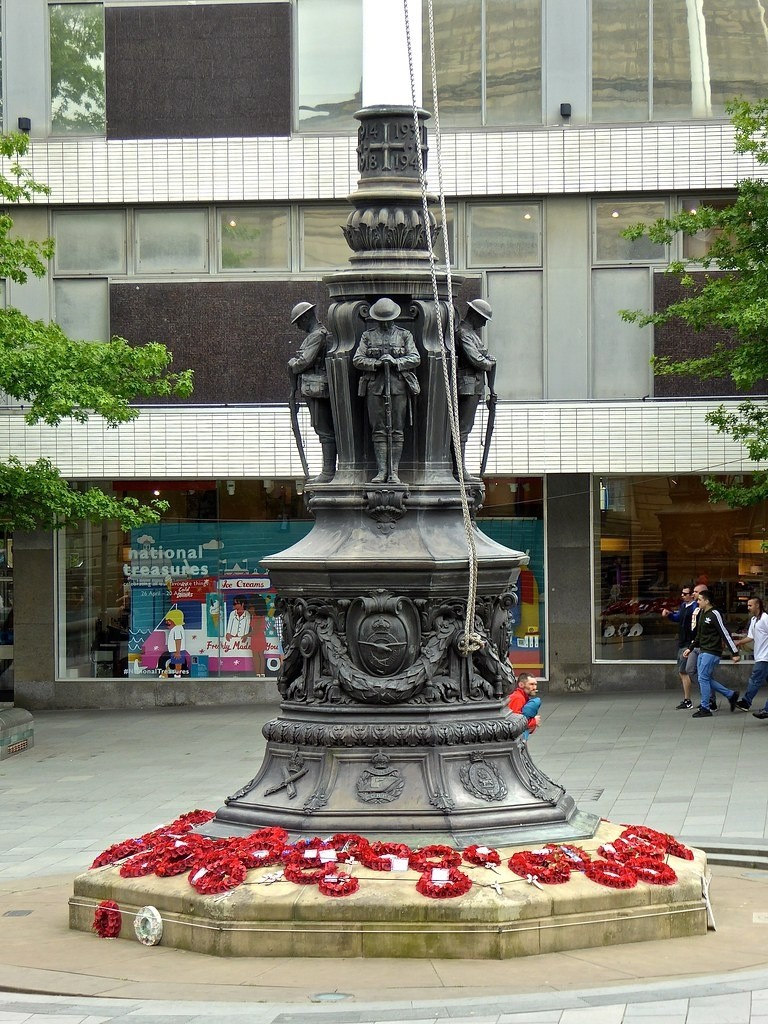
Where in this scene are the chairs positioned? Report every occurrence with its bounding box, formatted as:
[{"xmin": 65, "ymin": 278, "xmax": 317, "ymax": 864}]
[{"xmin": 731, "ymin": 633, "xmax": 754, "ymax": 660}]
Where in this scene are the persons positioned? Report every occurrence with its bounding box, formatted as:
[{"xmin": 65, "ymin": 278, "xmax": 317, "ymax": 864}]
[
  {"xmin": 507, "ymin": 672, "xmax": 541, "ymax": 741},
  {"xmin": 733, "ymin": 596, "xmax": 768, "ymax": 719},
  {"xmin": 682, "ymin": 590, "xmax": 741, "ymax": 718},
  {"xmin": 662, "ymin": 584, "xmax": 725, "ymax": 712}
]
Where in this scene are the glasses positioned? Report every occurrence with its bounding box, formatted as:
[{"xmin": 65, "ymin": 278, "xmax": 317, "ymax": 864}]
[{"xmin": 681, "ymin": 592, "xmax": 690, "ymax": 596}]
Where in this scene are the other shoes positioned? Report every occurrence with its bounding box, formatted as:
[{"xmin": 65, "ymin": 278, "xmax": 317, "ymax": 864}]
[
  {"xmin": 676, "ymin": 698, "xmax": 693, "ymax": 710},
  {"xmin": 736, "ymin": 701, "xmax": 750, "ymax": 711},
  {"xmin": 728, "ymin": 691, "xmax": 740, "ymax": 711},
  {"xmin": 753, "ymin": 708, "xmax": 768, "ymax": 719},
  {"xmin": 698, "ymin": 704, "xmax": 718, "ymax": 712},
  {"xmin": 692, "ymin": 709, "xmax": 712, "ymax": 717}
]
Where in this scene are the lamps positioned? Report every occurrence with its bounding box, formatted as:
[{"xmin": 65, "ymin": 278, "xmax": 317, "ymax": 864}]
[
  {"xmin": 263, "ymin": 480, "xmax": 271, "ymax": 488},
  {"xmin": 18, "ymin": 117, "xmax": 30, "ymax": 131},
  {"xmin": 561, "ymin": 103, "xmax": 571, "ymax": 117},
  {"xmin": 296, "ymin": 480, "xmax": 304, "ymax": 495},
  {"xmin": 227, "ymin": 481, "xmax": 235, "ymax": 495},
  {"xmin": 510, "ymin": 483, "xmax": 518, "ymax": 492}
]
